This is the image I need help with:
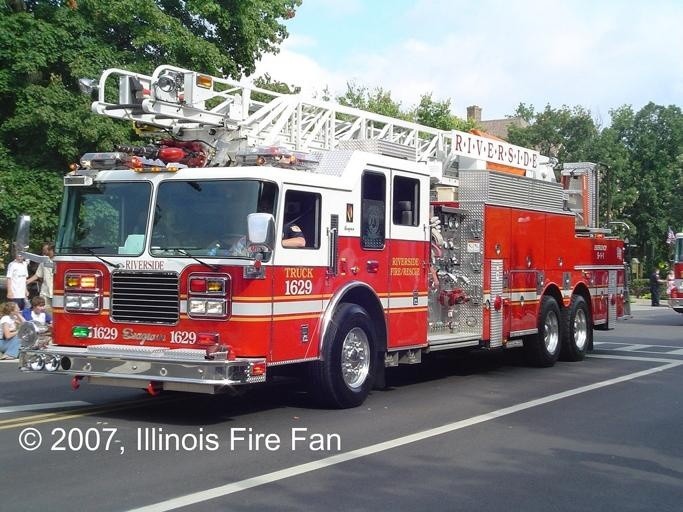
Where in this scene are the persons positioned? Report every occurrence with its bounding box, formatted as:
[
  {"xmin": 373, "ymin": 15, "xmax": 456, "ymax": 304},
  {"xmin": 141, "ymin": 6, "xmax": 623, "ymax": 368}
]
[
  {"xmin": 647, "ymin": 266, "xmax": 665, "ymax": 308},
  {"xmin": 0, "ymin": 301, "xmax": 25, "ymax": 362},
  {"xmin": 21, "ymin": 296, "xmax": 51, "ymax": 327},
  {"xmin": 204, "ymin": 197, "xmax": 306, "ymax": 256},
  {"xmin": 4, "ymin": 250, "xmax": 30, "ymax": 309},
  {"xmin": 25, "ymin": 243, "xmax": 53, "ymax": 301}
]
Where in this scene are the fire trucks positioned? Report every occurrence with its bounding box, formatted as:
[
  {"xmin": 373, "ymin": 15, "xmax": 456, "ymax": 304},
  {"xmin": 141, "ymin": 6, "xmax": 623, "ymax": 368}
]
[
  {"xmin": 15, "ymin": 64, "xmax": 632, "ymax": 409},
  {"xmin": 665, "ymin": 233, "xmax": 683, "ymax": 313}
]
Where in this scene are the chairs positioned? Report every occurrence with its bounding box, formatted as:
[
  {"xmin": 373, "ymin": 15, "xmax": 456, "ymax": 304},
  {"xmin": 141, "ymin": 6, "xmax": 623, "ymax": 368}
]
[{"xmin": 285, "ymin": 213, "xmax": 314, "ymax": 246}]
